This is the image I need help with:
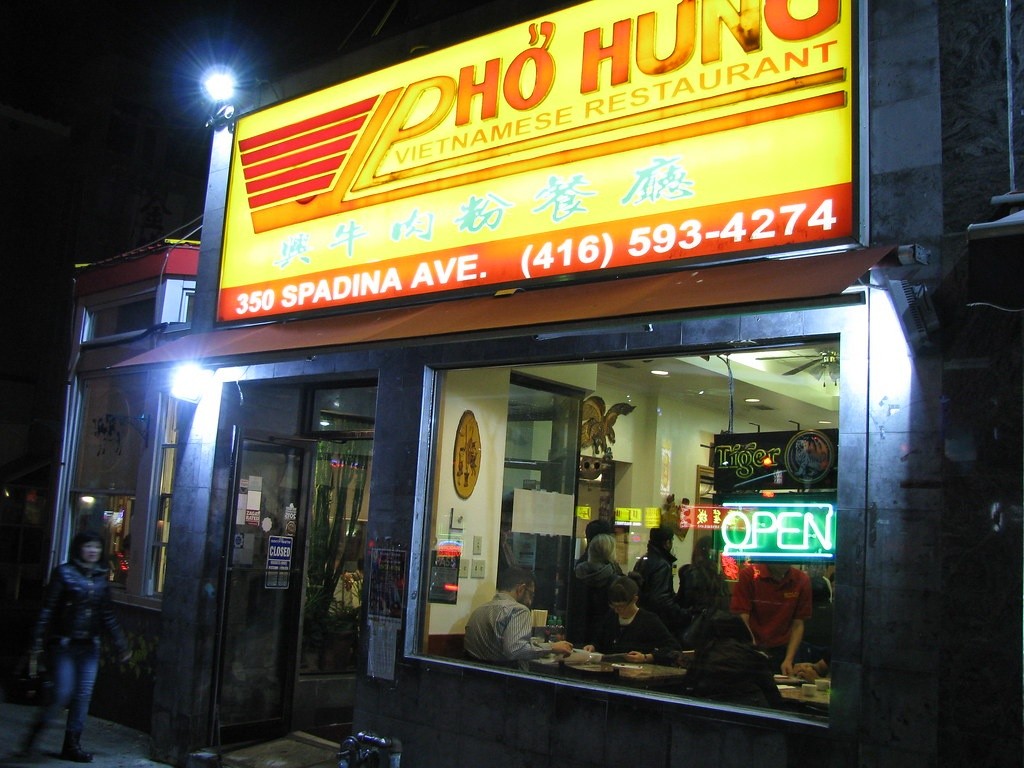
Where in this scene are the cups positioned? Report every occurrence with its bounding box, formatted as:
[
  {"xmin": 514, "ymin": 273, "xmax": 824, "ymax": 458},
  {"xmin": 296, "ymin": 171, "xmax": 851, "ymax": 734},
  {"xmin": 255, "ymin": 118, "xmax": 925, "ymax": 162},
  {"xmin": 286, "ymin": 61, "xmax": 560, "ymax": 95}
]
[
  {"xmin": 589, "ymin": 653, "xmax": 603, "ymax": 664},
  {"xmin": 802, "ymin": 684, "xmax": 817, "ymax": 696},
  {"xmin": 815, "ymin": 680, "xmax": 830, "ymax": 691}
]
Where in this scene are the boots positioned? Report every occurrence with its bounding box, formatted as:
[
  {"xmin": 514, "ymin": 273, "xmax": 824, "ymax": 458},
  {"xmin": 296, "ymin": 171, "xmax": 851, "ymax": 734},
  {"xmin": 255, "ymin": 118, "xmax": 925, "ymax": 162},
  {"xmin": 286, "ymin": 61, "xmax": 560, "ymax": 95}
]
[
  {"xmin": 60, "ymin": 730, "xmax": 94, "ymax": 762},
  {"xmin": 19, "ymin": 719, "xmax": 57, "ymax": 760}
]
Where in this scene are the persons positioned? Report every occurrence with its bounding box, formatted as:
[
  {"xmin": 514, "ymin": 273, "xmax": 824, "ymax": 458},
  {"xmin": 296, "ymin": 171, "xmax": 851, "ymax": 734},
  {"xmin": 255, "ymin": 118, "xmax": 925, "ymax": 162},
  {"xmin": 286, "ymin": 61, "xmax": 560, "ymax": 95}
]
[
  {"xmin": 14, "ymin": 530, "xmax": 132, "ymax": 762},
  {"xmin": 463, "ymin": 518, "xmax": 835, "ymax": 709}
]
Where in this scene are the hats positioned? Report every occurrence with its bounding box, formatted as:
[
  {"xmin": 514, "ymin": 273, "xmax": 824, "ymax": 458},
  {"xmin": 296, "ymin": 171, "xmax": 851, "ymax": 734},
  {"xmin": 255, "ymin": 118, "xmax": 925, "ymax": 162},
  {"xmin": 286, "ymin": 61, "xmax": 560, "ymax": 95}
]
[
  {"xmin": 584, "ymin": 518, "xmax": 615, "ymax": 543},
  {"xmin": 649, "ymin": 527, "xmax": 674, "ymax": 541}
]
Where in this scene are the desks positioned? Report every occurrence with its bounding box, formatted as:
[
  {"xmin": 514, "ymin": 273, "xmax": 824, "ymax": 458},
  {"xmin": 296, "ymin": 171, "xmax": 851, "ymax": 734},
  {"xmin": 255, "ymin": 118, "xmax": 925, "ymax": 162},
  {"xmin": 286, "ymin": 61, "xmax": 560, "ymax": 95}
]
[
  {"xmin": 774, "ymin": 673, "xmax": 832, "ymax": 714},
  {"xmin": 532, "ymin": 650, "xmax": 687, "ymax": 690}
]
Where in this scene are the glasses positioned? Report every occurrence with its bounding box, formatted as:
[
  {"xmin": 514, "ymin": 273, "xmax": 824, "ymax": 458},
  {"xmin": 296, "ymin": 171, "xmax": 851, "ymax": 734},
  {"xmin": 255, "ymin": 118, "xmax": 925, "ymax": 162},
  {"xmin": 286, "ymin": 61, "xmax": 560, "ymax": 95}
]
[
  {"xmin": 519, "ymin": 583, "xmax": 536, "ymax": 599},
  {"xmin": 608, "ymin": 599, "xmax": 633, "ymax": 611}
]
